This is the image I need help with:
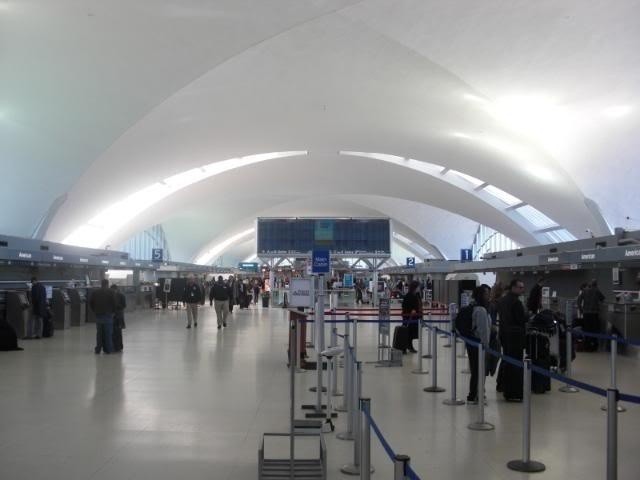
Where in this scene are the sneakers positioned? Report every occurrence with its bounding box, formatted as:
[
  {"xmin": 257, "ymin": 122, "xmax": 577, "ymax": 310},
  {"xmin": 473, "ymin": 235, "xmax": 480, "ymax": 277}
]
[
  {"xmin": 401, "ymin": 348, "xmax": 417, "ymax": 354},
  {"xmin": 218, "ymin": 323, "xmax": 226, "ymax": 328},
  {"xmin": 467, "ymin": 392, "xmax": 487, "ymax": 404},
  {"xmin": 187, "ymin": 323, "xmax": 197, "ymax": 328},
  {"xmin": 21, "ymin": 335, "xmax": 41, "ymax": 339}
]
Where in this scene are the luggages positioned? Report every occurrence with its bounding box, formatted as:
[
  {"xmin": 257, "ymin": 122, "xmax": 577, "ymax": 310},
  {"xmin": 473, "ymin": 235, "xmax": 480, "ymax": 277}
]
[
  {"xmin": 393, "ymin": 315, "xmax": 414, "ymax": 349},
  {"xmin": 502, "ymin": 360, "xmax": 523, "ymax": 400},
  {"xmin": 102, "ymin": 323, "xmax": 122, "ymax": 353},
  {"xmin": 529, "ymin": 327, "xmax": 551, "ymax": 393},
  {"xmin": 43, "ymin": 318, "xmax": 54, "ymax": 337}
]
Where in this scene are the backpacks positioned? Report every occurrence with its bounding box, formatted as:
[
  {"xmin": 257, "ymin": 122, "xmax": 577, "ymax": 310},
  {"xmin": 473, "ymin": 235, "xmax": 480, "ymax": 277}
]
[{"xmin": 454, "ymin": 304, "xmax": 490, "ymax": 336}]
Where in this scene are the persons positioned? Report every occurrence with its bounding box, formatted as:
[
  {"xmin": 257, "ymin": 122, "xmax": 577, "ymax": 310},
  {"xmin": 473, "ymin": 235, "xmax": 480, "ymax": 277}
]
[
  {"xmin": 21, "ymin": 276, "xmax": 48, "ymax": 340},
  {"xmin": 455, "ymin": 279, "xmax": 544, "ymax": 402},
  {"xmin": 401, "ymin": 280, "xmax": 421, "ymax": 354},
  {"xmin": 327, "ymin": 272, "xmax": 373, "ymax": 304},
  {"xmin": 182, "ymin": 272, "xmax": 262, "ymax": 329},
  {"xmin": 89, "ymin": 278, "xmax": 119, "ymax": 354},
  {"xmin": 577, "ymin": 279, "xmax": 606, "ymax": 351},
  {"xmin": 101, "ymin": 282, "xmax": 127, "ymax": 352},
  {"xmin": 378, "ymin": 274, "xmax": 432, "ymax": 298}
]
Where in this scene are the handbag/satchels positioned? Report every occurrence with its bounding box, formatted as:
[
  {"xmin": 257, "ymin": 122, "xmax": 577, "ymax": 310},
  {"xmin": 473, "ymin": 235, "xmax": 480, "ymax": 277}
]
[{"xmin": 532, "ymin": 309, "xmax": 557, "ymax": 327}]
